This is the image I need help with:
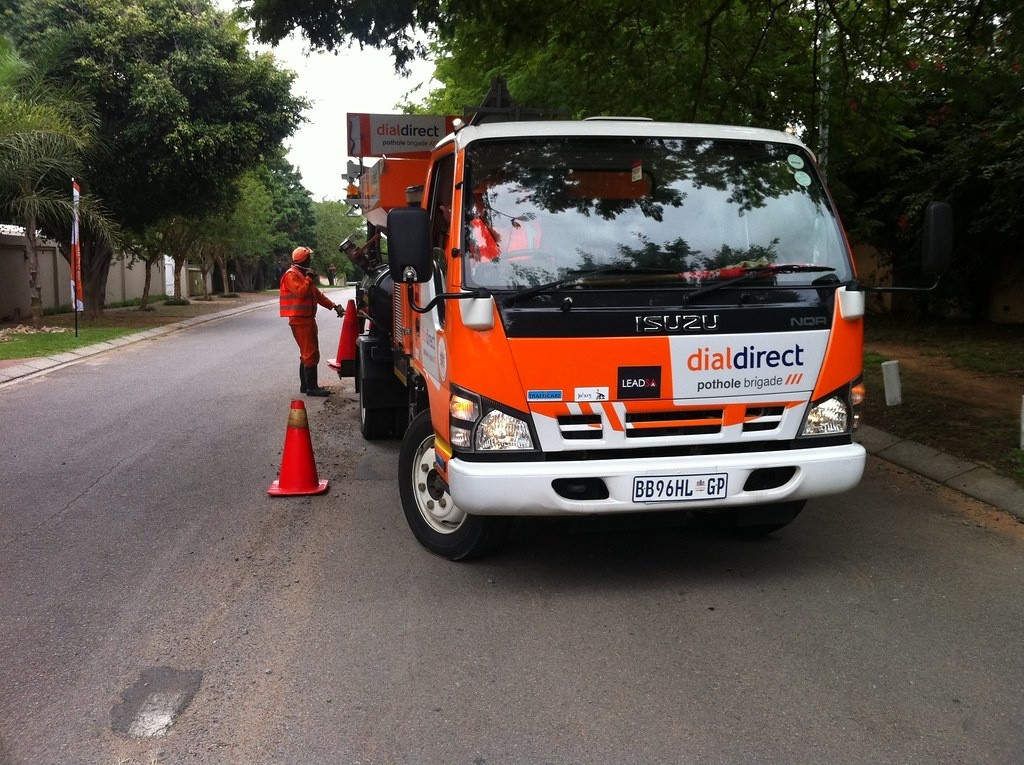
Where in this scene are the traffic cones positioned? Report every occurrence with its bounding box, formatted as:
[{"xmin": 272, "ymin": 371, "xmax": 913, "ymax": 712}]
[
  {"xmin": 326, "ymin": 299, "xmax": 361, "ymax": 372},
  {"xmin": 267, "ymin": 400, "xmax": 329, "ymax": 496}
]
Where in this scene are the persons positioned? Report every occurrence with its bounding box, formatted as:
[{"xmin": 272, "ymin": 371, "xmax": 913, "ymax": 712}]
[
  {"xmin": 439, "ymin": 173, "xmax": 552, "ymax": 267},
  {"xmin": 280, "ymin": 247, "xmax": 336, "ymax": 395},
  {"xmin": 328, "ymin": 269, "xmax": 334, "ymax": 285}
]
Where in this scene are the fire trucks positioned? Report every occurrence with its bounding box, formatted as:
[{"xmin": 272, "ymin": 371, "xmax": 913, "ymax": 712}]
[{"xmin": 338, "ymin": 73, "xmax": 954, "ymax": 560}]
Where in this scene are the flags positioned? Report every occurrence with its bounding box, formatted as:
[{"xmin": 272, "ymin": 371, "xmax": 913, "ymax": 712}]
[{"xmin": 71, "ymin": 180, "xmax": 84, "ymax": 312}]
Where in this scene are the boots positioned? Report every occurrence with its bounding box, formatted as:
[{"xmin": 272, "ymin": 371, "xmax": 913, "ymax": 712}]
[
  {"xmin": 304, "ymin": 364, "xmax": 331, "ymax": 397},
  {"xmin": 299, "ymin": 361, "xmax": 325, "ymax": 394}
]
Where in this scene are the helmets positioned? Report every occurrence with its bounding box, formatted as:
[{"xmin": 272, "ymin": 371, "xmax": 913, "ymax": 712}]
[{"xmin": 291, "ymin": 246, "xmax": 309, "ymax": 264}]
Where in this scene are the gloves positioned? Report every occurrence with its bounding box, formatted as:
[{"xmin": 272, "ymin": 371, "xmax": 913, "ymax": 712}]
[
  {"xmin": 335, "ymin": 304, "xmax": 344, "ymax": 317},
  {"xmin": 306, "ymin": 268, "xmax": 316, "ymax": 276}
]
